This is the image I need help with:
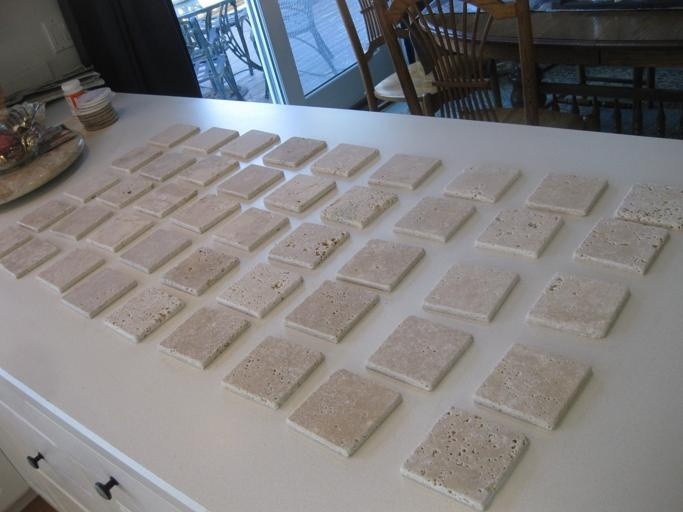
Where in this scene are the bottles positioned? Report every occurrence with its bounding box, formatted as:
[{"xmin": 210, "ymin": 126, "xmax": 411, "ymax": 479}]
[{"xmin": 61, "ymin": 79, "xmax": 85, "ymax": 112}]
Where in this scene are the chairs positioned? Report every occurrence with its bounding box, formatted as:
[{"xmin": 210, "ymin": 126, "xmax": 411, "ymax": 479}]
[
  {"xmin": 338, "ymin": 0, "xmax": 682, "ymax": 139},
  {"xmin": 179, "ymin": 0, "xmax": 338, "ymax": 105}
]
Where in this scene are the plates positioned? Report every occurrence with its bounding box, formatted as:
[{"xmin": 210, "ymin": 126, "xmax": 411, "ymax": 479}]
[{"xmin": 72, "ymin": 86, "xmax": 120, "ymax": 132}]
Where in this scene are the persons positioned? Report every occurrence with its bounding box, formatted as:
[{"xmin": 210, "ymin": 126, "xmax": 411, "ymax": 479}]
[{"xmin": 385, "ymin": 0, "xmax": 554, "ymax": 83}]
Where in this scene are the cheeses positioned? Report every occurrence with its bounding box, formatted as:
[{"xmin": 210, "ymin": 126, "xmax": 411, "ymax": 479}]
[{"xmin": 0, "ymin": 124, "xmax": 683, "ymax": 512}]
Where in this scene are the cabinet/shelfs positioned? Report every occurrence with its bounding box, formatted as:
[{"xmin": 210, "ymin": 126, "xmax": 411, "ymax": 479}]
[{"xmin": 0, "ymin": 374, "xmax": 189, "ymax": 511}]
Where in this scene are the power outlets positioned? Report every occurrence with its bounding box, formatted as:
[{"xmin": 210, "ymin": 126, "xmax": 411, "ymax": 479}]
[{"xmin": 40, "ymin": 13, "xmax": 73, "ymax": 56}]
